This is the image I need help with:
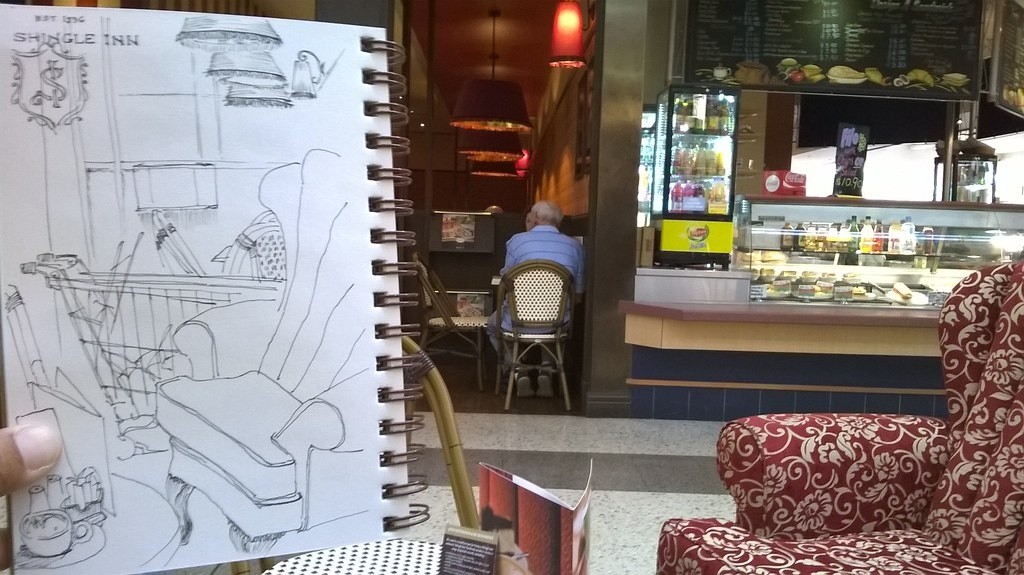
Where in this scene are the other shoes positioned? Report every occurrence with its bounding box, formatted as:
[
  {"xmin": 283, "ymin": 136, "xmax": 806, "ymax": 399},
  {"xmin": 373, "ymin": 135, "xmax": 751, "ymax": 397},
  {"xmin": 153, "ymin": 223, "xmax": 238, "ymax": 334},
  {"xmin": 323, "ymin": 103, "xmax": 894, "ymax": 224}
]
[
  {"xmin": 536, "ymin": 368, "xmax": 553, "ymax": 396},
  {"xmin": 516, "ymin": 368, "xmax": 535, "ymax": 397}
]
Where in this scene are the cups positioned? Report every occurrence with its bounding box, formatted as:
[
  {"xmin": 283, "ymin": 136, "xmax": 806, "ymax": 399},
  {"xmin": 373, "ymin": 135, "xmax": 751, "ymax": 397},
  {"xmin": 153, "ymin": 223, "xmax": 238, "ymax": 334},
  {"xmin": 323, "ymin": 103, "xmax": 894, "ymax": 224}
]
[{"xmin": 712, "ymin": 66, "xmax": 731, "ymax": 81}]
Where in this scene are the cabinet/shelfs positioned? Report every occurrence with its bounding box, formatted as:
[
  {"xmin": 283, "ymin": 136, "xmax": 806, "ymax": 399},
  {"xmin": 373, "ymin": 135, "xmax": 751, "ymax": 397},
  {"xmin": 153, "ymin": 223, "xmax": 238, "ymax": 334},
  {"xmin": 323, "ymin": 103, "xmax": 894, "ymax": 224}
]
[
  {"xmin": 740, "ymin": 199, "xmax": 1024, "ymax": 317},
  {"xmin": 650, "ymin": 82, "xmax": 740, "ymax": 272}
]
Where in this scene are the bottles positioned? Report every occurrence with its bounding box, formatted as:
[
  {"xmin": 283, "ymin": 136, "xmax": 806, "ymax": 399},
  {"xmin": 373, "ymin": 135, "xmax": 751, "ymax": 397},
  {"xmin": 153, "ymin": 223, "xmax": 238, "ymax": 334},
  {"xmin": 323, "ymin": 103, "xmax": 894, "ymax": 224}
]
[
  {"xmin": 663, "ymin": 93, "xmax": 729, "ymax": 213},
  {"xmin": 781, "ymin": 215, "xmax": 917, "ymax": 255}
]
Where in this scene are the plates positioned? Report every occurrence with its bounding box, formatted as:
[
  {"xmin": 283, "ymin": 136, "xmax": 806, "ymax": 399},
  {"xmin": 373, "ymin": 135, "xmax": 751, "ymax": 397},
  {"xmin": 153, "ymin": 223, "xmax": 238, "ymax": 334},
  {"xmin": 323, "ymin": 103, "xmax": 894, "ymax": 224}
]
[
  {"xmin": 825, "ymin": 73, "xmax": 868, "ymax": 85},
  {"xmin": 765, "ymin": 284, "xmax": 930, "ymax": 307}
]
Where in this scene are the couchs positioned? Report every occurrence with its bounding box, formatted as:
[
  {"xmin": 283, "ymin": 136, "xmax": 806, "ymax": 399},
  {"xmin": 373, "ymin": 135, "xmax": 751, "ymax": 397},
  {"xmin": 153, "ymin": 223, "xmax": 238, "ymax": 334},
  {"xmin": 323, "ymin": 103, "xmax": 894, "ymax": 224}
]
[{"xmin": 655, "ymin": 257, "xmax": 1024, "ymax": 575}]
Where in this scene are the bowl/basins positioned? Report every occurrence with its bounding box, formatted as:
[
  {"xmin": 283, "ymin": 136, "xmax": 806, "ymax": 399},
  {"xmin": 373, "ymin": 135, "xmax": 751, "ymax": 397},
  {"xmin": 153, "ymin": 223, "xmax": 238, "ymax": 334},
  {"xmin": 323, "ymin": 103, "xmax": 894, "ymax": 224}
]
[
  {"xmin": 745, "ymin": 273, "xmax": 862, "ymax": 284},
  {"xmin": 777, "ymin": 64, "xmax": 822, "ymax": 84}
]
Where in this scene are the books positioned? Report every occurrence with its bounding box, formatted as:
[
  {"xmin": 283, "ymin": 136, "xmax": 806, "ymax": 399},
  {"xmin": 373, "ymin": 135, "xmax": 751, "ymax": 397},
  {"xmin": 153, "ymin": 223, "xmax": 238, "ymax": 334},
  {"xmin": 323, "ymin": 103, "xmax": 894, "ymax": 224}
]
[{"xmin": 1, "ymin": 0, "xmax": 431, "ymax": 575}]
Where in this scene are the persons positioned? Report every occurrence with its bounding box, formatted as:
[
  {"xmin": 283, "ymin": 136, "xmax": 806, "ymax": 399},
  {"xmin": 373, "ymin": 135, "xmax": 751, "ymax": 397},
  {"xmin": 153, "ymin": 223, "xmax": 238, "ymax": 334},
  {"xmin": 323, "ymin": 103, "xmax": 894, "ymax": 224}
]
[
  {"xmin": 483, "ymin": 201, "xmax": 590, "ymax": 398},
  {"xmin": 523, "ymin": 206, "xmax": 542, "ymax": 233},
  {"xmin": 2, "ymin": 397, "xmax": 63, "ymax": 575}
]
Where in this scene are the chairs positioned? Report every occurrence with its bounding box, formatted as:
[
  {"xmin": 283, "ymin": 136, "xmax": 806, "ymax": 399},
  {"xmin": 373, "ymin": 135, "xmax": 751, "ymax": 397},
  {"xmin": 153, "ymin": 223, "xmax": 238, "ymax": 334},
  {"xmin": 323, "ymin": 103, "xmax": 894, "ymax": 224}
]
[
  {"xmin": 497, "ymin": 258, "xmax": 581, "ymax": 412},
  {"xmin": 410, "ymin": 250, "xmax": 497, "ymax": 390}
]
